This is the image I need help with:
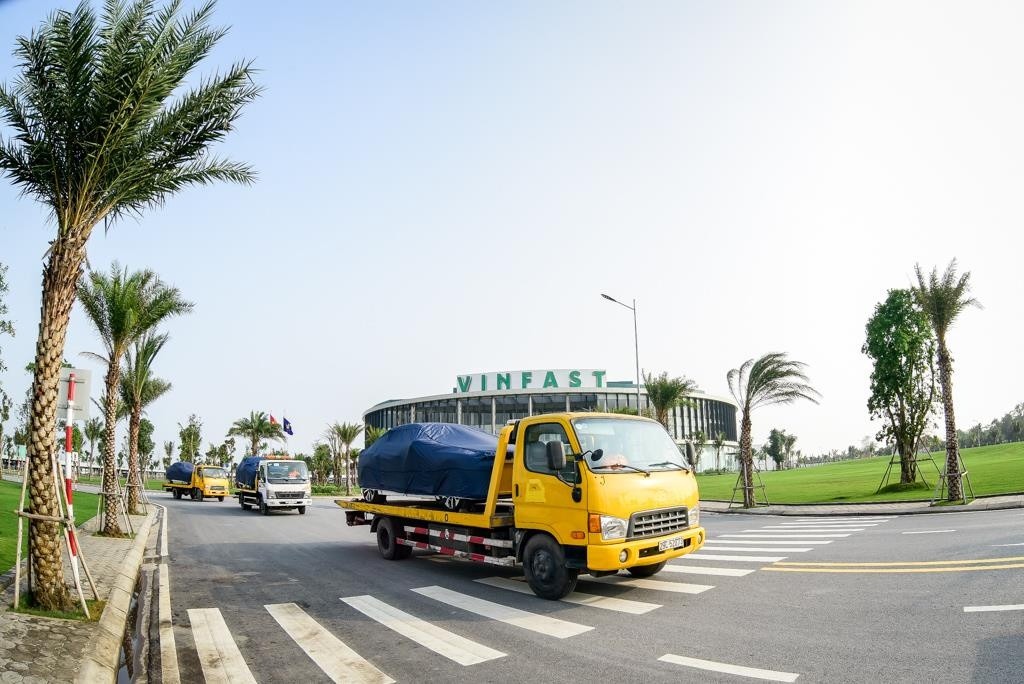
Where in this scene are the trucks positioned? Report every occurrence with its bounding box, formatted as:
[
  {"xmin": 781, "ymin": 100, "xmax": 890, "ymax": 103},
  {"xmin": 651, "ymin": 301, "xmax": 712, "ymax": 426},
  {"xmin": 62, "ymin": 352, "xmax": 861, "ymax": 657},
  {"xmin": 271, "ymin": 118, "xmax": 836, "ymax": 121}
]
[
  {"xmin": 332, "ymin": 411, "xmax": 706, "ymax": 600},
  {"xmin": 161, "ymin": 464, "xmax": 230, "ymax": 502},
  {"xmin": 232, "ymin": 454, "xmax": 312, "ymax": 515}
]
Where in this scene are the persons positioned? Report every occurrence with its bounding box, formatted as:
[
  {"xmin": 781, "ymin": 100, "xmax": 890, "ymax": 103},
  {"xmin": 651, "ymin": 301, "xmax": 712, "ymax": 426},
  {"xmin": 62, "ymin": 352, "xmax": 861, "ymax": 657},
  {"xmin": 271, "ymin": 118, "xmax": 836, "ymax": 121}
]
[
  {"xmin": 604, "ymin": 440, "xmax": 627, "ymax": 470},
  {"xmin": 288, "ymin": 467, "xmax": 300, "ymax": 477}
]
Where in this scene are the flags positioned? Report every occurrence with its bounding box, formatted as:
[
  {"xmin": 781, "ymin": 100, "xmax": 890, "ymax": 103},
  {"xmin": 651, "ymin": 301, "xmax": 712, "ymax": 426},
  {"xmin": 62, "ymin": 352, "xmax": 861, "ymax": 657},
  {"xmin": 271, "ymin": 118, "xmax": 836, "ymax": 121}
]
[
  {"xmin": 284, "ymin": 417, "xmax": 293, "ymax": 435},
  {"xmin": 270, "ymin": 416, "xmax": 277, "ymax": 424}
]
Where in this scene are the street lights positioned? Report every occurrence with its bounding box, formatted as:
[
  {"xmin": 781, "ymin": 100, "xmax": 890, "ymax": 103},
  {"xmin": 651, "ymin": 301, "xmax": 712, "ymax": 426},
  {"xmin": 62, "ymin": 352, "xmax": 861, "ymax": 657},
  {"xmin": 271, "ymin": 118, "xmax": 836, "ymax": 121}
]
[{"xmin": 601, "ymin": 293, "xmax": 642, "ymax": 418}]
